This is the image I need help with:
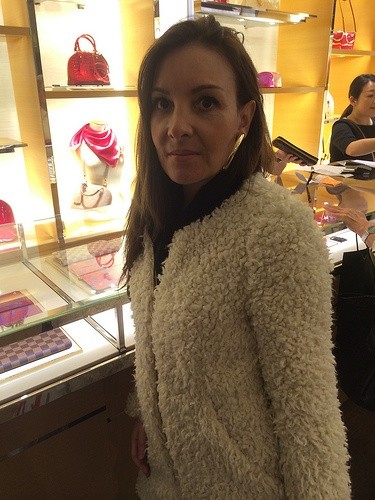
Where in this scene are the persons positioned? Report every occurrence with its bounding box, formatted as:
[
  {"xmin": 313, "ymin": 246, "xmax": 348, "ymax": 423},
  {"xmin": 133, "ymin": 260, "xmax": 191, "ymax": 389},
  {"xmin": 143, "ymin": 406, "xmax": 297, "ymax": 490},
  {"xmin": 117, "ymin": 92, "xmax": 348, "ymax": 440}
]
[
  {"xmin": 322, "ymin": 200, "xmax": 375, "ymax": 258},
  {"xmin": 265, "ymin": 148, "xmax": 302, "ymax": 177},
  {"xmin": 330, "ymin": 74, "xmax": 375, "ymax": 164},
  {"xmin": 122, "ymin": 14, "xmax": 354, "ymax": 500}
]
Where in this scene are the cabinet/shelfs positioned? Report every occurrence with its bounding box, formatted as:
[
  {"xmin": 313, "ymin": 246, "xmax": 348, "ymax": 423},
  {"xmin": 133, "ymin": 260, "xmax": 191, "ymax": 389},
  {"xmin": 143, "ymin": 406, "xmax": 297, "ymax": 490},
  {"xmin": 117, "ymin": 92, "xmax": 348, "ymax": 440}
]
[{"xmin": 0, "ymin": 0, "xmax": 375, "ymax": 500}]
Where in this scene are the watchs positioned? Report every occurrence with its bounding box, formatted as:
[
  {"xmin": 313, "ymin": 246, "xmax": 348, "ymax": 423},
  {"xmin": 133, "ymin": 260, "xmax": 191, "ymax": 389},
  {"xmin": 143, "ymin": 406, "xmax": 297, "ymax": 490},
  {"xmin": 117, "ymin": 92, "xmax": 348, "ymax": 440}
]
[{"xmin": 362, "ymin": 225, "xmax": 375, "ymax": 243}]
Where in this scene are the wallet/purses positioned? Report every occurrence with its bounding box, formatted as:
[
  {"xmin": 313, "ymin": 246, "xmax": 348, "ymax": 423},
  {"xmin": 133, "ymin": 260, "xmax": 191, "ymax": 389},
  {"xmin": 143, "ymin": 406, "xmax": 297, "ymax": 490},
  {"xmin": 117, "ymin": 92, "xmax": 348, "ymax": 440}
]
[
  {"xmin": 0, "ymin": 290, "xmax": 43, "ymax": 326},
  {"xmin": 272, "ymin": 136, "xmax": 319, "ymax": 167},
  {"xmin": 0, "ymin": 327, "xmax": 72, "ymax": 375},
  {"xmin": 257, "ymin": 71, "xmax": 274, "ymax": 87},
  {"xmin": 271, "ymin": 72, "xmax": 283, "ymax": 88},
  {"xmin": 53, "ymin": 241, "xmax": 122, "ymax": 292}
]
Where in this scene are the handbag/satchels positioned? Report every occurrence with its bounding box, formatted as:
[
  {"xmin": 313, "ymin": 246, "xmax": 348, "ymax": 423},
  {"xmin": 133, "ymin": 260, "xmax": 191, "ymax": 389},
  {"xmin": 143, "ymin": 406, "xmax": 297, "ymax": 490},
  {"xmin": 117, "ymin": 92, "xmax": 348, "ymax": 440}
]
[
  {"xmin": 0, "ymin": 200, "xmax": 18, "ymax": 241},
  {"xmin": 68, "ymin": 34, "xmax": 110, "ymax": 85},
  {"xmin": 70, "ymin": 180, "xmax": 112, "ymax": 209},
  {"xmin": 333, "ymin": 0, "xmax": 357, "ymax": 50},
  {"xmin": 87, "ymin": 237, "xmax": 122, "ymax": 268},
  {"xmin": 333, "ymin": 220, "xmax": 375, "ymax": 413}
]
